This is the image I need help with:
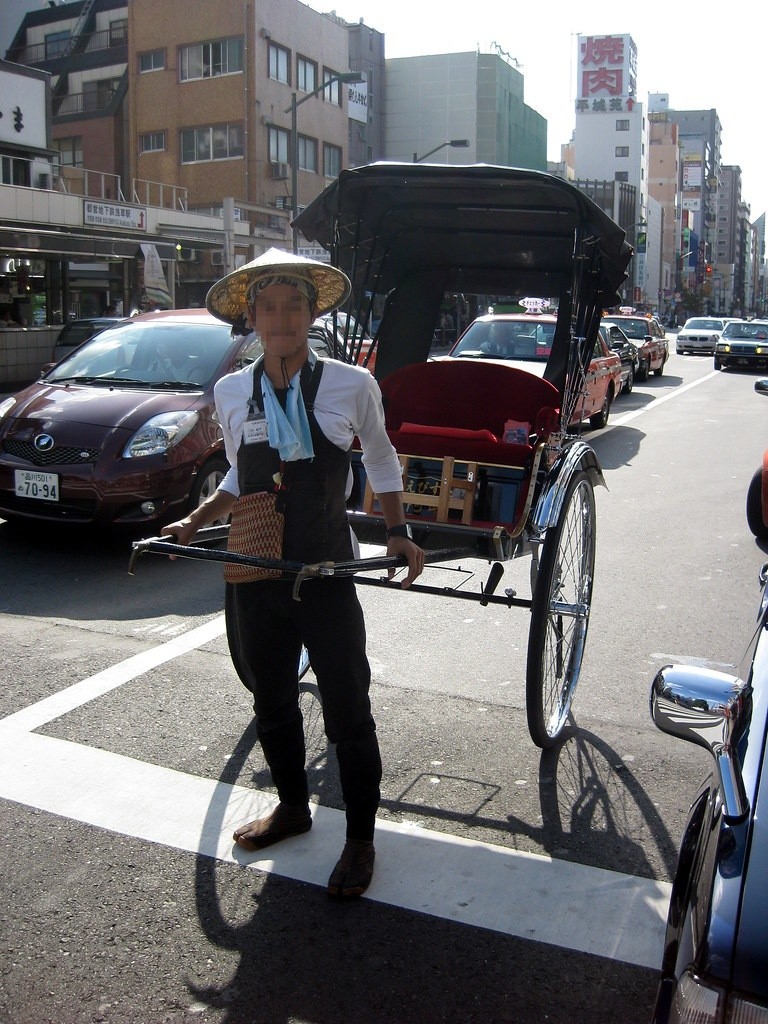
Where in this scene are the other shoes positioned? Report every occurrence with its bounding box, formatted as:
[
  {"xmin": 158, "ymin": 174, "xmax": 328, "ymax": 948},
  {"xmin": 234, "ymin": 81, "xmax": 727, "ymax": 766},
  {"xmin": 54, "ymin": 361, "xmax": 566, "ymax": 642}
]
[
  {"xmin": 233, "ymin": 795, "xmax": 312, "ymax": 850},
  {"xmin": 327, "ymin": 838, "xmax": 375, "ymax": 898}
]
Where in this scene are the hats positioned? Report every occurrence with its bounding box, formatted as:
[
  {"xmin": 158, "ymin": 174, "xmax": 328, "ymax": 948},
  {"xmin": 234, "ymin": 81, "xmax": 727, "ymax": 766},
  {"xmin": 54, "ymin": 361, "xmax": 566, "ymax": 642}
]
[{"xmin": 206, "ymin": 247, "xmax": 352, "ymax": 337}]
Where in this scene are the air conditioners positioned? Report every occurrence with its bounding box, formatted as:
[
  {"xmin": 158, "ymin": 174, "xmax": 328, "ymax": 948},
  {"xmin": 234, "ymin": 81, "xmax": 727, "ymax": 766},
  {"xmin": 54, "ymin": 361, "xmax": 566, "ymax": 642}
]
[
  {"xmin": 211, "ymin": 248, "xmax": 230, "ymax": 265},
  {"xmin": 178, "ymin": 248, "xmax": 196, "ymax": 262},
  {"xmin": 273, "ymin": 162, "xmax": 290, "ymax": 179},
  {"xmin": 263, "ymin": 27, "xmax": 272, "ymax": 39}
]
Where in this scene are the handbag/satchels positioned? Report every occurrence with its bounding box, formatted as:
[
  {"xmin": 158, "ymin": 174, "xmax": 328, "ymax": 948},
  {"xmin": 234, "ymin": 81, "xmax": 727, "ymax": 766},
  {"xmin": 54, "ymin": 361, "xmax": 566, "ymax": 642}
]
[{"xmin": 223, "ymin": 485, "xmax": 287, "ymax": 585}]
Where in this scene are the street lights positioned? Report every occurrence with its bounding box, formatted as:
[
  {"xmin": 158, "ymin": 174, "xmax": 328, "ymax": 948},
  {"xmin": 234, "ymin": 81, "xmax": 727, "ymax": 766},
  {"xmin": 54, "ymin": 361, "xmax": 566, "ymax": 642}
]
[
  {"xmin": 718, "ymin": 272, "xmax": 736, "ymax": 312},
  {"xmin": 285, "ymin": 71, "xmax": 368, "ymax": 257}
]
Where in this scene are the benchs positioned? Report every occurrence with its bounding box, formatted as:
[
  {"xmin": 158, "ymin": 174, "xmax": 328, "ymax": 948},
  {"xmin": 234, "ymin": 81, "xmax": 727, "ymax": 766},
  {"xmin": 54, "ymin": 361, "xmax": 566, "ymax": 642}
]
[{"xmin": 348, "ymin": 359, "xmax": 561, "ymax": 530}]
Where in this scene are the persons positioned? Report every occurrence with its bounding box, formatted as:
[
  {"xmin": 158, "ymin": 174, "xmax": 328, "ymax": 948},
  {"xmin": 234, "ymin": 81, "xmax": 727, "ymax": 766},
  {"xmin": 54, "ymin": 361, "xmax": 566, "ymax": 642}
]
[
  {"xmin": 480, "ymin": 325, "xmax": 513, "ymax": 354},
  {"xmin": 161, "ymin": 246, "xmax": 426, "ymax": 898}
]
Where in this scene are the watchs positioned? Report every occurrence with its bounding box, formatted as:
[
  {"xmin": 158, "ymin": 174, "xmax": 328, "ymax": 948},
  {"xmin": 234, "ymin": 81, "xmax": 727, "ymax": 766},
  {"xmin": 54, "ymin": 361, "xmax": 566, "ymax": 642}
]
[{"xmin": 386, "ymin": 523, "xmax": 412, "ymax": 540}]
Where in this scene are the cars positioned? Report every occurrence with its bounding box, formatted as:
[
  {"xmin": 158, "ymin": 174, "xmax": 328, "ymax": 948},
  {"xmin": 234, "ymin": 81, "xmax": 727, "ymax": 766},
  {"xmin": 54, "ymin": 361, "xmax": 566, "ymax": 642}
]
[
  {"xmin": 600, "ymin": 306, "xmax": 670, "ymax": 383},
  {"xmin": 713, "ymin": 321, "xmax": 768, "ymax": 373},
  {"xmin": 318, "ymin": 312, "xmax": 377, "ymax": 377},
  {"xmin": 445, "ymin": 297, "xmax": 622, "ymax": 429},
  {"xmin": 649, "ymin": 557, "xmax": 768, "ymax": 1024},
  {"xmin": 719, "ymin": 317, "xmax": 768, "ymax": 334},
  {"xmin": 674, "ymin": 316, "xmax": 724, "ymax": 355},
  {"xmin": 52, "ymin": 316, "xmax": 145, "ymax": 376},
  {"xmin": 0, "ymin": 306, "xmax": 360, "ymax": 529},
  {"xmin": 599, "ymin": 322, "xmax": 640, "ymax": 394}
]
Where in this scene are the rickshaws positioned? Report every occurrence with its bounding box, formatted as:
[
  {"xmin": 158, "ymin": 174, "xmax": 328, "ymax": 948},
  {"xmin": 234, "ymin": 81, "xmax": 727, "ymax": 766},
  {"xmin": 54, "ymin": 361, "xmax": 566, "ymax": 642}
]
[{"xmin": 126, "ymin": 160, "xmax": 640, "ymax": 750}]
